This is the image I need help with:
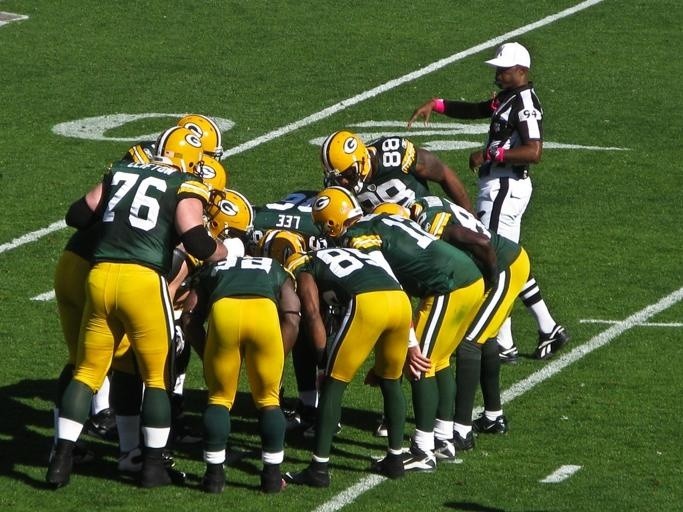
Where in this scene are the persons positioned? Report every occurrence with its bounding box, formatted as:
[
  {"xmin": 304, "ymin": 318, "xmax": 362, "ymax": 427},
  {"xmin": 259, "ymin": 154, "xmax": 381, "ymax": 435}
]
[
  {"xmin": 44, "ymin": 113, "xmax": 530, "ymax": 495},
  {"xmin": 408, "ymin": 42, "xmax": 570, "ymax": 364}
]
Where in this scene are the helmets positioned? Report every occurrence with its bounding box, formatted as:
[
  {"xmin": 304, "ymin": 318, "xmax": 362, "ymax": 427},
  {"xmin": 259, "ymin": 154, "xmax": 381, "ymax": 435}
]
[
  {"xmin": 321, "ymin": 130, "xmax": 371, "ymax": 189},
  {"xmin": 156, "ymin": 126, "xmax": 203, "ymax": 177},
  {"xmin": 209, "ymin": 189, "xmax": 254, "ymax": 239},
  {"xmin": 312, "ymin": 186, "xmax": 363, "ymax": 237},
  {"xmin": 201, "ymin": 156, "xmax": 226, "ymax": 222},
  {"xmin": 370, "ymin": 203, "xmax": 411, "ymax": 219},
  {"xmin": 258, "ymin": 229, "xmax": 307, "ymax": 265},
  {"xmin": 177, "ymin": 114, "xmax": 223, "ymax": 162}
]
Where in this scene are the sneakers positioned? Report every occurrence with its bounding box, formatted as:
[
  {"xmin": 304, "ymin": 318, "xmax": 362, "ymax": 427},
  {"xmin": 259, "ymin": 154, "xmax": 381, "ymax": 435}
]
[
  {"xmin": 303, "ymin": 422, "xmax": 342, "ymax": 437},
  {"xmin": 472, "ymin": 411, "xmax": 508, "ymax": 434},
  {"xmin": 376, "ymin": 420, "xmax": 388, "ymax": 437},
  {"xmin": 285, "ymin": 458, "xmax": 330, "ymax": 487},
  {"xmin": 286, "ymin": 411, "xmax": 312, "ymax": 434},
  {"xmin": 402, "ymin": 440, "xmax": 437, "ymax": 470},
  {"xmin": 498, "ymin": 344, "xmax": 519, "ymax": 362},
  {"xmin": 88, "ymin": 407, "xmax": 119, "ymax": 434},
  {"xmin": 201, "ymin": 463, "xmax": 225, "ymax": 494},
  {"xmin": 261, "ymin": 463, "xmax": 281, "ymax": 493},
  {"xmin": 532, "ymin": 324, "xmax": 569, "ymax": 360},
  {"xmin": 435, "ymin": 436, "xmax": 456, "ymax": 459},
  {"xmin": 46, "ymin": 438, "xmax": 76, "ymax": 486},
  {"xmin": 140, "ymin": 446, "xmax": 186, "ymax": 488},
  {"xmin": 49, "ymin": 443, "xmax": 96, "ymax": 465},
  {"xmin": 373, "ymin": 452, "xmax": 404, "ymax": 479},
  {"xmin": 453, "ymin": 429, "xmax": 475, "ymax": 450},
  {"xmin": 118, "ymin": 448, "xmax": 176, "ymax": 480}
]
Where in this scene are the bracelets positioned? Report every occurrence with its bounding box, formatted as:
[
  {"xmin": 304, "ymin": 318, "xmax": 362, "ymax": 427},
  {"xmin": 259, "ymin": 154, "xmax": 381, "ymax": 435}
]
[{"xmin": 431, "ymin": 99, "xmax": 445, "ymax": 114}]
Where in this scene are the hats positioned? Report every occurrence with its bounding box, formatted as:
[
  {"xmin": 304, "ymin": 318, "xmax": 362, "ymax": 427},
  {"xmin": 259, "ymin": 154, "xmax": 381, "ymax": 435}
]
[{"xmin": 485, "ymin": 42, "xmax": 531, "ymax": 69}]
